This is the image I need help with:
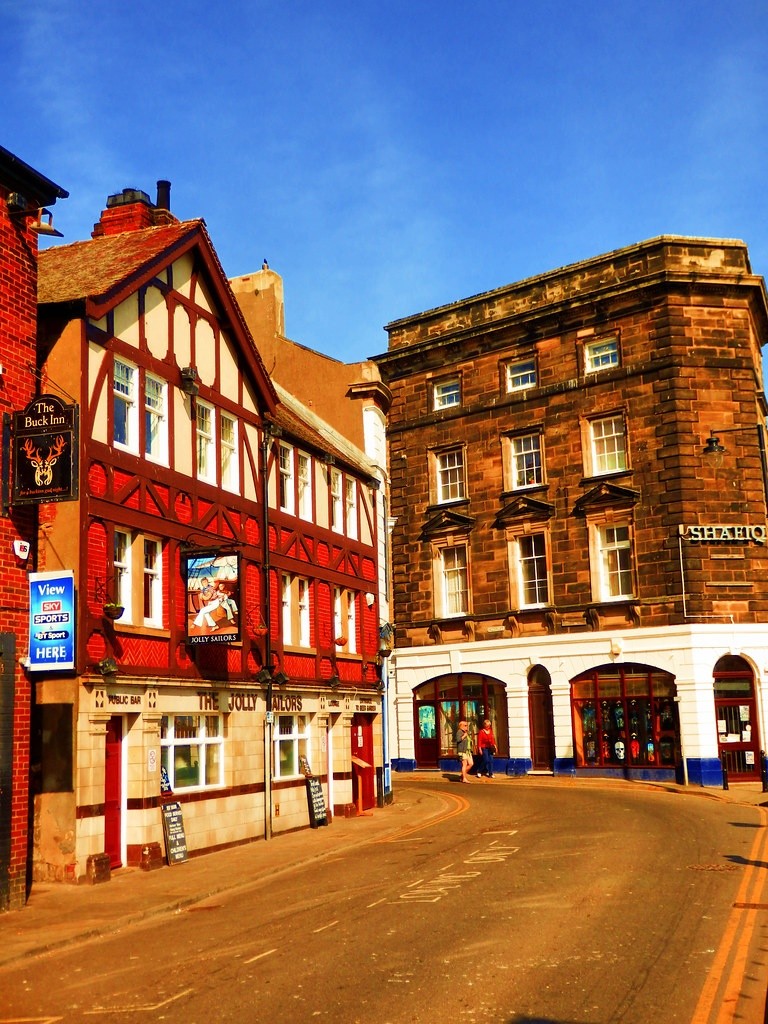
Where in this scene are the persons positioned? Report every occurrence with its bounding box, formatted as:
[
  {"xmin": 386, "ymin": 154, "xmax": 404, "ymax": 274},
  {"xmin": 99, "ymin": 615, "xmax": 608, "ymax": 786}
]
[
  {"xmin": 476, "ymin": 720, "xmax": 497, "ymax": 778},
  {"xmin": 456, "ymin": 721, "xmax": 474, "ymax": 783},
  {"xmin": 760, "ymin": 750, "xmax": 768, "ymax": 792}
]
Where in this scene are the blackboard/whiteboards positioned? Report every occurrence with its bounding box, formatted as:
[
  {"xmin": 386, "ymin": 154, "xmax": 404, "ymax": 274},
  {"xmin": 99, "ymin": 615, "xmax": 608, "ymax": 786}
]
[
  {"xmin": 163, "ymin": 808, "xmax": 188, "ymax": 866},
  {"xmin": 307, "ymin": 777, "xmax": 327, "ymax": 822}
]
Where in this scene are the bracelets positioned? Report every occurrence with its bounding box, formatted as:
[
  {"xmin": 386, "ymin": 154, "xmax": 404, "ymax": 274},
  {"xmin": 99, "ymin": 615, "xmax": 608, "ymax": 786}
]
[{"xmin": 479, "ymin": 749, "xmax": 481, "ymax": 751}]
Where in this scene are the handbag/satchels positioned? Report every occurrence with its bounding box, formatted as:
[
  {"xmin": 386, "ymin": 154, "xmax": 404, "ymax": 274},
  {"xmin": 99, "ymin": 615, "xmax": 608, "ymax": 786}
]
[
  {"xmin": 488, "ymin": 746, "xmax": 496, "ymax": 755},
  {"xmin": 455, "ymin": 755, "xmax": 462, "ymax": 764}
]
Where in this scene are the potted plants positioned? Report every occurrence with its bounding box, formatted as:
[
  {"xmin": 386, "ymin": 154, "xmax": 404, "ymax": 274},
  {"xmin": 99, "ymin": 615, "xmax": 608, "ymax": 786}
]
[
  {"xmin": 526, "ymin": 471, "xmax": 534, "ymax": 484},
  {"xmin": 252, "ymin": 625, "xmax": 268, "ymax": 636},
  {"xmin": 334, "ymin": 636, "xmax": 347, "ymax": 647},
  {"xmin": 375, "ymin": 646, "xmax": 392, "ymax": 665},
  {"xmin": 105, "ymin": 603, "xmax": 126, "ymax": 619}
]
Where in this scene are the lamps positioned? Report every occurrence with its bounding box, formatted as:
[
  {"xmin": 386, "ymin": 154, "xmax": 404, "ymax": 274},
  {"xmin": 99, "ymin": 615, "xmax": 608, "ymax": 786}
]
[
  {"xmin": 368, "ymin": 480, "xmax": 380, "ymax": 490},
  {"xmin": 268, "ymin": 421, "xmax": 282, "ymax": 436},
  {"xmin": 9, "ymin": 208, "xmax": 64, "ymax": 238},
  {"xmin": 322, "ymin": 454, "xmax": 336, "ymax": 465},
  {"xmin": 255, "ymin": 668, "xmax": 290, "ymax": 685},
  {"xmin": 99, "ymin": 658, "xmax": 117, "ymax": 675},
  {"xmin": 372, "ymin": 680, "xmax": 385, "ymax": 690},
  {"xmin": 331, "ymin": 674, "xmax": 341, "ymax": 689},
  {"xmin": 182, "ymin": 366, "xmax": 199, "ymax": 396}
]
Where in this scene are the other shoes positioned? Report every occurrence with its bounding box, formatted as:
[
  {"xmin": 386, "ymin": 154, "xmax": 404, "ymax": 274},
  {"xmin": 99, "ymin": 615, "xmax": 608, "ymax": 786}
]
[
  {"xmin": 486, "ymin": 773, "xmax": 494, "ymax": 778},
  {"xmin": 477, "ymin": 770, "xmax": 481, "ymax": 777}
]
